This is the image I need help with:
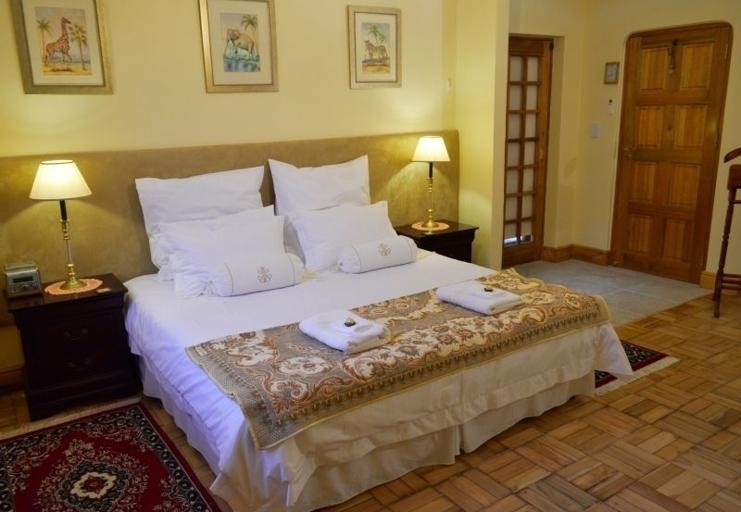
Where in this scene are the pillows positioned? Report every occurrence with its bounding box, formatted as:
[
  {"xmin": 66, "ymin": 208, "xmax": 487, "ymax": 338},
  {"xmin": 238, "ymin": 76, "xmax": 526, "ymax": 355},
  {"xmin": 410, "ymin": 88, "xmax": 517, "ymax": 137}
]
[
  {"xmin": 210, "ymin": 252, "xmax": 308, "ymax": 299},
  {"xmin": 286, "ymin": 199, "xmax": 400, "ymax": 274},
  {"xmin": 135, "ymin": 164, "xmax": 266, "ymax": 269},
  {"xmin": 266, "ymin": 152, "xmax": 372, "ymax": 269},
  {"xmin": 336, "ymin": 235, "xmax": 420, "ymax": 274},
  {"xmin": 156, "ymin": 204, "xmax": 290, "ymax": 298}
]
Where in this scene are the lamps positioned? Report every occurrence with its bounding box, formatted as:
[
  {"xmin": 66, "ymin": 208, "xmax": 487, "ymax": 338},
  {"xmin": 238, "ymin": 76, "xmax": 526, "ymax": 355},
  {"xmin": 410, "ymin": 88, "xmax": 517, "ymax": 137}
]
[
  {"xmin": 28, "ymin": 158, "xmax": 103, "ymax": 295},
  {"xmin": 410, "ymin": 133, "xmax": 452, "ymax": 234}
]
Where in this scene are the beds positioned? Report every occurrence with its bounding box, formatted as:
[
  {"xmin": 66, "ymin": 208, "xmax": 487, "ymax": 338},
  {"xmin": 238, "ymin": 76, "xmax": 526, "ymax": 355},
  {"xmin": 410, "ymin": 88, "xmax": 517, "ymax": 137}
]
[{"xmin": 117, "ymin": 237, "xmax": 635, "ymax": 511}]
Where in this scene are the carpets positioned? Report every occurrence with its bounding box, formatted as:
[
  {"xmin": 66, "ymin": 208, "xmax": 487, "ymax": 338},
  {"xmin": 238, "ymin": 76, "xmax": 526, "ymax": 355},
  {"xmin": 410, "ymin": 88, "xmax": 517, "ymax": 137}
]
[
  {"xmin": 512, "ymin": 255, "xmax": 717, "ymax": 331},
  {"xmin": 0, "ymin": 401, "xmax": 222, "ymax": 512},
  {"xmin": 594, "ymin": 336, "xmax": 682, "ymax": 394}
]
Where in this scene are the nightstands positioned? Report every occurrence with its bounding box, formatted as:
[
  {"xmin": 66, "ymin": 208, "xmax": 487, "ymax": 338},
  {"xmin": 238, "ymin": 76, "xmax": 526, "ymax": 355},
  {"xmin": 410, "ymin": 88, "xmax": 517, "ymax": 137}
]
[
  {"xmin": 394, "ymin": 218, "xmax": 480, "ymax": 262},
  {"xmin": 2, "ymin": 268, "xmax": 150, "ymax": 424}
]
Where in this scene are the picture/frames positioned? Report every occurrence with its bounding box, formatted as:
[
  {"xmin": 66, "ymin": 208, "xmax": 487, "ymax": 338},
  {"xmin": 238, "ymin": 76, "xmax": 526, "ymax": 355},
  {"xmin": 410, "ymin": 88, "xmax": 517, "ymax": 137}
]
[
  {"xmin": 603, "ymin": 61, "xmax": 621, "ymax": 86},
  {"xmin": 345, "ymin": 5, "xmax": 404, "ymax": 93},
  {"xmin": 6, "ymin": 0, "xmax": 113, "ymax": 98},
  {"xmin": 197, "ymin": 1, "xmax": 282, "ymax": 95}
]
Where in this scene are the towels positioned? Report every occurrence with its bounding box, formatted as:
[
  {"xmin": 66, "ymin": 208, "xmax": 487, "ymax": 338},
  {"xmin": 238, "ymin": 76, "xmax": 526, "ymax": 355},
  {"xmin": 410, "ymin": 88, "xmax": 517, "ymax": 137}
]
[
  {"xmin": 297, "ymin": 304, "xmax": 395, "ymax": 357},
  {"xmin": 433, "ymin": 275, "xmax": 528, "ymax": 319}
]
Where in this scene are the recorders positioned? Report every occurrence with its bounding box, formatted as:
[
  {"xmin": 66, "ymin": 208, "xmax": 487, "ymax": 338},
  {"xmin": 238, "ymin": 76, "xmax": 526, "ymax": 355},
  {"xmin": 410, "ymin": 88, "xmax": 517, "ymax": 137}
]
[{"xmin": 5, "ymin": 266, "xmax": 42, "ymax": 299}]
